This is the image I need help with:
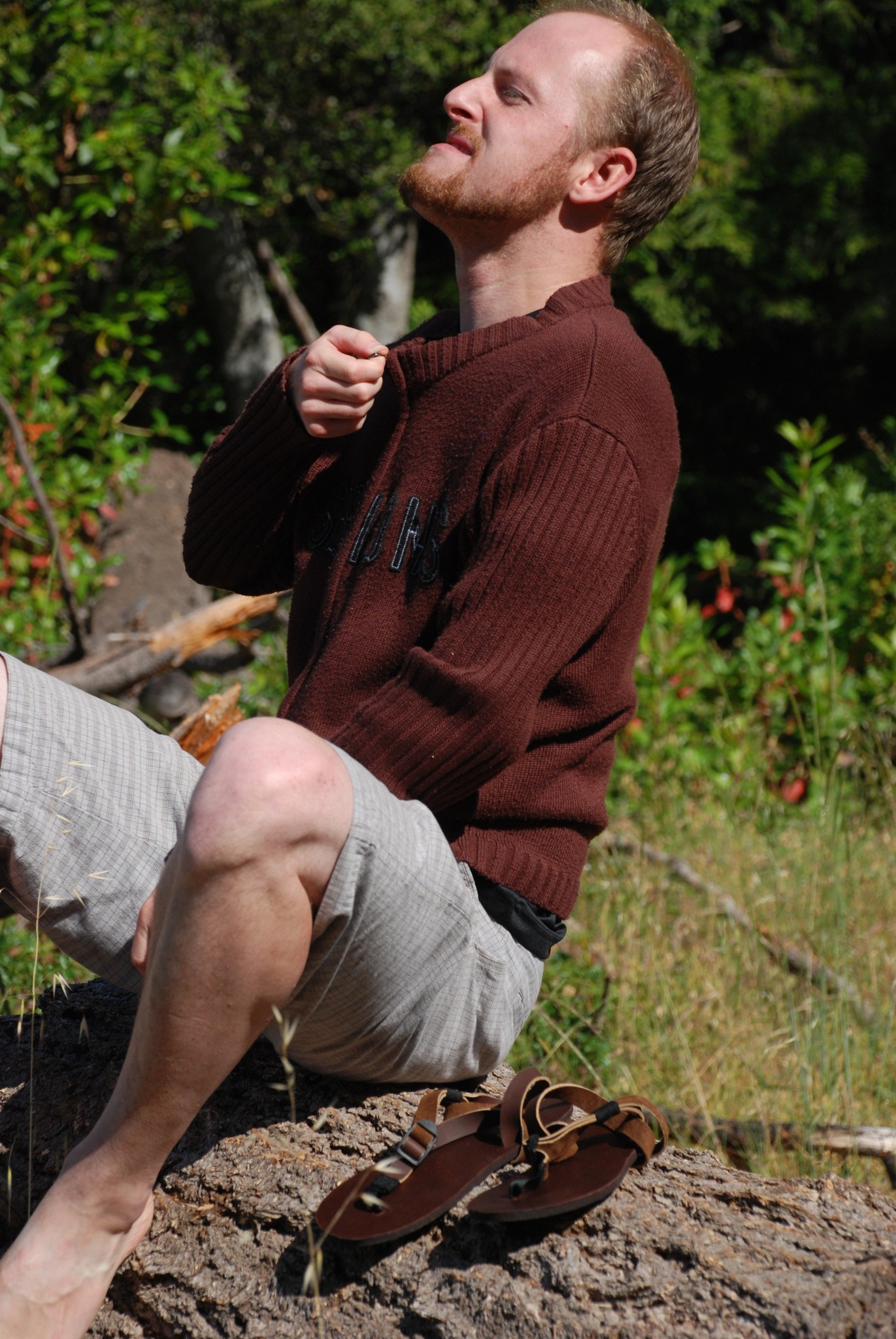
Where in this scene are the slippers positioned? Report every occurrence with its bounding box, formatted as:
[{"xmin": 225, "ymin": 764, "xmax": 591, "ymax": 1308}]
[
  {"xmin": 466, "ymin": 1083, "xmax": 671, "ymax": 1221},
  {"xmin": 314, "ymin": 1071, "xmax": 572, "ymax": 1248}
]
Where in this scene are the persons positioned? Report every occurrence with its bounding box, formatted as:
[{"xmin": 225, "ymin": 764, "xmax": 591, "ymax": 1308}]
[{"xmin": 0, "ymin": 0, "xmax": 702, "ymax": 1339}]
